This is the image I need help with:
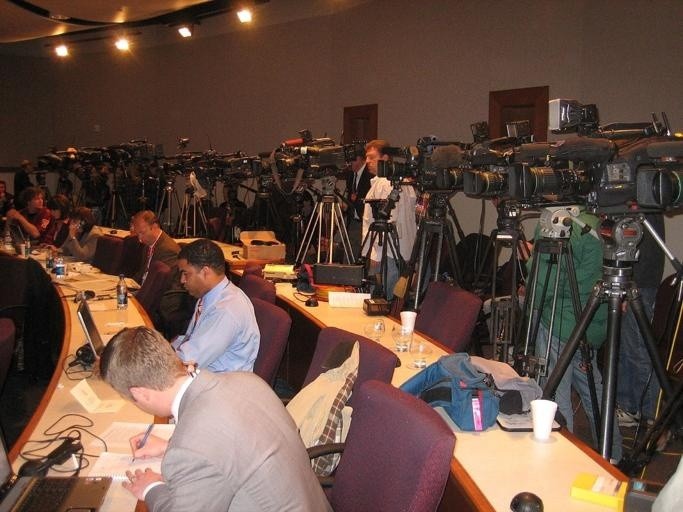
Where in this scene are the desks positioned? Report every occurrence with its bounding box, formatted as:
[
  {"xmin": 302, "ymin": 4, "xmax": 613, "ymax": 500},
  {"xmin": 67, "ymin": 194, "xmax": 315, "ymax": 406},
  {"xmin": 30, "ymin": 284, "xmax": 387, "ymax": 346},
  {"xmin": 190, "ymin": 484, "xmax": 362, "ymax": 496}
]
[
  {"xmin": 1, "ymin": 237, "xmax": 173, "ymax": 511},
  {"xmin": 92, "ymin": 225, "xmax": 655, "ymax": 512}
]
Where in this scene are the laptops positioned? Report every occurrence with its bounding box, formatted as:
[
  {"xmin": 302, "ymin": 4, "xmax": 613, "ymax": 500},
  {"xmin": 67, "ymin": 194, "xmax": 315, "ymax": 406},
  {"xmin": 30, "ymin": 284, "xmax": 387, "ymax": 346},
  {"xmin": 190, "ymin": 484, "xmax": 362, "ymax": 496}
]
[
  {"xmin": 78, "ymin": 299, "xmax": 123, "ymax": 360},
  {"xmin": 0, "ymin": 420, "xmax": 112, "ymax": 512}
]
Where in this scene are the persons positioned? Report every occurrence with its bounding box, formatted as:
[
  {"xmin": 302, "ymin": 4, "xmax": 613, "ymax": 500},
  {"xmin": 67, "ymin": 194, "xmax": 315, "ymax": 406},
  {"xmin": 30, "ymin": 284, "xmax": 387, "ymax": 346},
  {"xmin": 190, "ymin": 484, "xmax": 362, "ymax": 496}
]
[{"xmin": 0, "ymin": 140, "xmax": 666, "ymax": 511}]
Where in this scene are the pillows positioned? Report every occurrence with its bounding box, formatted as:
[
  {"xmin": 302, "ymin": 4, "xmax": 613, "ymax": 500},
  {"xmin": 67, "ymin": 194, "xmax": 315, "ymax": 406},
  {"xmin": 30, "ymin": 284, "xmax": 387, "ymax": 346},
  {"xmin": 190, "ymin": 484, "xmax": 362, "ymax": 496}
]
[{"xmin": 285, "ymin": 340, "xmax": 360, "ymax": 477}]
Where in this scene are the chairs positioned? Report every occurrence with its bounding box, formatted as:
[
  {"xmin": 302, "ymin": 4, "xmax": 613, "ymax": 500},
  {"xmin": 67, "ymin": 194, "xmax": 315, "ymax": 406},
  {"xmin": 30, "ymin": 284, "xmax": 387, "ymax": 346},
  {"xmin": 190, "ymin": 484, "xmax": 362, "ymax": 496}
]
[
  {"xmin": 2, "ymin": 317, "xmax": 16, "ymax": 387},
  {"xmin": 302, "ymin": 380, "xmax": 456, "ymax": 511},
  {"xmin": 412, "ymin": 280, "xmax": 483, "ymax": 355},
  {"xmin": 236, "ymin": 274, "xmax": 276, "ymax": 304},
  {"xmin": 250, "ymin": 297, "xmax": 293, "ymax": 388},
  {"xmin": 298, "ymin": 326, "xmax": 401, "ymax": 441},
  {"xmin": 241, "ymin": 261, "xmax": 262, "ymax": 284},
  {"xmin": 91, "ymin": 236, "xmax": 124, "ymax": 275},
  {"xmin": 135, "ymin": 259, "xmax": 172, "ymax": 311}
]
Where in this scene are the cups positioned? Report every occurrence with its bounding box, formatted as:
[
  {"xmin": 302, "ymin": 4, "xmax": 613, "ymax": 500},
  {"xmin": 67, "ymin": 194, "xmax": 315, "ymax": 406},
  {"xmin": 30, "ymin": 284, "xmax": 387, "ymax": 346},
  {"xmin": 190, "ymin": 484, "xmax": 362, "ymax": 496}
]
[
  {"xmin": 527, "ymin": 398, "xmax": 559, "ymax": 443},
  {"xmin": 367, "ymin": 310, "xmax": 433, "ymax": 370}
]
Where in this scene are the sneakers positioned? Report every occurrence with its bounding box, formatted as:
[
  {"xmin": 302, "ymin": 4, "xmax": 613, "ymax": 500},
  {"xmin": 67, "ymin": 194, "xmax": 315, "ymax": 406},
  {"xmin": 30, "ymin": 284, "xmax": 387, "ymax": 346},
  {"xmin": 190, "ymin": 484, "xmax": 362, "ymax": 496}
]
[{"xmin": 614, "ymin": 407, "xmax": 657, "ymax": 428}]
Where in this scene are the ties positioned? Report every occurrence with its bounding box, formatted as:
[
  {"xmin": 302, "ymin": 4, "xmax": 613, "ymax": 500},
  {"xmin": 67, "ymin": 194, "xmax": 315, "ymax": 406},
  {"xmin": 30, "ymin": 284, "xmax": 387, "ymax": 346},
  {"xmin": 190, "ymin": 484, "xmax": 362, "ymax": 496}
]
[
  {"xmin": 176, "ymin": 297, "xmax": 206, "ymax": 353},
  {"xmin": 353, "ymin": 172, "xmax": 357, "ymax": 192}
]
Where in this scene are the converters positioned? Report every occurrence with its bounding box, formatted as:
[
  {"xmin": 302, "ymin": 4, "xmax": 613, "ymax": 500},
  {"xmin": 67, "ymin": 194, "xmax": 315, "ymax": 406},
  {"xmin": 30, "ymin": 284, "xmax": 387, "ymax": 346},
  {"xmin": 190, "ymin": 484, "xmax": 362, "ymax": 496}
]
[{"xmin": 47, "ymin": 438, "xmax": 81, "ymax": 465}]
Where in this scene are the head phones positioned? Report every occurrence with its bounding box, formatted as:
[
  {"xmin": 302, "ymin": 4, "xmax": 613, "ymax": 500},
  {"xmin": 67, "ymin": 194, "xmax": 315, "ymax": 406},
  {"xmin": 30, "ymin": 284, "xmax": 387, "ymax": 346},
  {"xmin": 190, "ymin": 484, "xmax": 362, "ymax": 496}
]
[{"xmin": 69, "ymin": 343, "xmax": 95, "ymax": 367}]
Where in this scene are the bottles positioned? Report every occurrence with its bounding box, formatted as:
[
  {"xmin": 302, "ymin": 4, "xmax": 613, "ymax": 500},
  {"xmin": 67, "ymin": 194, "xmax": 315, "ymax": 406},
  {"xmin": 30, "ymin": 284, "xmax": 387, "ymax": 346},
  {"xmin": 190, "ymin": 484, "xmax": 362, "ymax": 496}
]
[
  {"xmin": 44, "ymin": 246, "xmax": 69, "ymax": 280},
  {"xmin": 116, "ymin": 273, "xmax": 127, "ymax": 311},
  {"xmin": 4, "ymin": 230, "xmax": 33, "ymax": 256}
]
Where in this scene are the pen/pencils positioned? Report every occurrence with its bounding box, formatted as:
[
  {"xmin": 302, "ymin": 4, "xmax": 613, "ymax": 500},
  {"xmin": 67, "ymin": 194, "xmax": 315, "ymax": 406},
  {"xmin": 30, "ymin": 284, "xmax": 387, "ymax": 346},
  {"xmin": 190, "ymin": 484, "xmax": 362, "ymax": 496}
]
[
  {"xmin": 131, "ymin": 421, "xmax": 156, "ymax": 463},
  {"xmin": 93, "ymin": 297, "xmax": 118, "ymax": 301}
]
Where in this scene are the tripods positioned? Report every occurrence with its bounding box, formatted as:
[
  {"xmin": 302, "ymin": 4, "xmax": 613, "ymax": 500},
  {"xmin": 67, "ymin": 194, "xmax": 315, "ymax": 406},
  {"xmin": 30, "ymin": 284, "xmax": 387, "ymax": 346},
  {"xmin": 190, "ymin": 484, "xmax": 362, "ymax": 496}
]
[
  {"xmin": 135, "ymin": 178, "xmax": 155, "ymax": 213},
  {"xmin": 174, "ymin": 186, "xmax": 216, "ymax": 240},
  {"xmin": 508, "ymin": 206, "xmax": 602, "ymax": 454},
  {"xmin": 202, "ymin": 179, "xmax": 216, "ymax": 240},
  {"xmin": 291, "ymin": 176, "xmax": 356, "ymax": 265},
  {"xmin": 290, "ymin": 180, "xmax": 309, "ymax": 266},
  {"xmin": 250, "ymin": 178, "xmax": 291, "ymax": 266},
  {"xmin": 104, "ymin": 189, "xmax": 131, "ymax": 230},
  {"xmin": 481, "ymin": 201, "xmax": 531, "ymax": 378},
  {"xmin": 357, "ymin": 223, "xmax": 473, "ymax": 313},
  {"xmin": 616, "ymin": 276, "xmax": 683, "ymax": 476},
  {"xmin": 541, "ymin": 215, "xmax": 683, "ymax": 466},
  {"xmin": 156, "ymin": 184, "xmax": 186, "ymax": 237},
  {"xmin": 219, "ymin": 189, "xmax": 249, "ymax": 247}
]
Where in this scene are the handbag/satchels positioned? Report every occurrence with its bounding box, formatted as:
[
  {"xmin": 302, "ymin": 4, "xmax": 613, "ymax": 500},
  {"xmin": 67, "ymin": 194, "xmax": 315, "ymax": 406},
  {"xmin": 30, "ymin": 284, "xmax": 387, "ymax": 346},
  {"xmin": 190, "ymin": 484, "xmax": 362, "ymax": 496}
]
[{"xmin": 399, "ymin": 351, "xmax": 544, "ymax": 433}]
